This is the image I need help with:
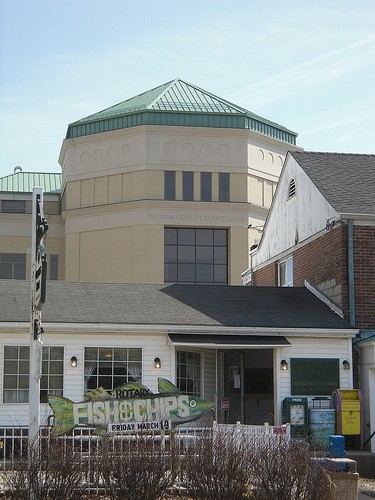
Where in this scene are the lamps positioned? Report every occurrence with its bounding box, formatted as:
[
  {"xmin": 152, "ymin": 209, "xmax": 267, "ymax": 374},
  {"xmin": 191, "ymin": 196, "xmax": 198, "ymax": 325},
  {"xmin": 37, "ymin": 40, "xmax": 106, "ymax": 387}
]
[
  {"xmin": 154, "ymin": 357, "xmax": 161, "ymax": 368},
  {"xmin": 70, "ymin": 356, "xmax": 77, "ymax": 367},
  {"xmin": 281, "ymin": 359, "xmax": 287, "ymax": 371},
  {"xmin": 343, "ymin": 360, "xmax": 350, "ymax": 370}
]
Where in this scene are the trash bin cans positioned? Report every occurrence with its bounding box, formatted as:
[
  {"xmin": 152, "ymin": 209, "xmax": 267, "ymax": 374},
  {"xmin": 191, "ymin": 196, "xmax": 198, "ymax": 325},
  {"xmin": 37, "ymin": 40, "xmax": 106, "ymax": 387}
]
[{"xmin": 305, "ymin": 457, "xmax": 359, "ymax": 500}]
